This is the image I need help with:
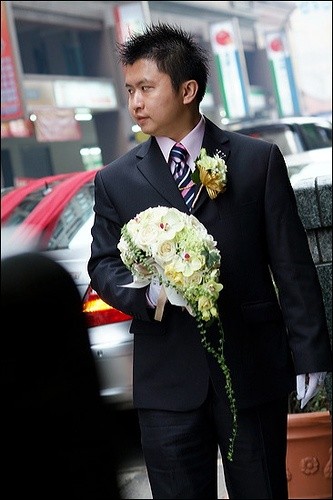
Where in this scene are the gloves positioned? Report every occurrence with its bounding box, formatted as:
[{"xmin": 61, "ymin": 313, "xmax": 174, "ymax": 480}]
[
  {"xmin": 147, "ymin": 272, "xmax": 162, "ymax": 306},
  {"xmin": 296, "ymin": 372, "xmax": 327, "ymax": 409}
]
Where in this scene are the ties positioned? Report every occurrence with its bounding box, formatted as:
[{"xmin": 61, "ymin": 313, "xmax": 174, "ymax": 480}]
[{"xmin": 170, "ymin": 143, "xmax": 198, "ymax": 213}]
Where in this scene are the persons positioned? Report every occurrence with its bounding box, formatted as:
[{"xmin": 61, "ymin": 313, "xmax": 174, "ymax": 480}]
[{"xmin": 87, "ymin": 19, "xmax": 333, "ymax": 500}]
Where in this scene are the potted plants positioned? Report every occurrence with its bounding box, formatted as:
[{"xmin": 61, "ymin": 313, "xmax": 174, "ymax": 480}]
[{"xmin": 283, "ymin": 376, "xmax": 333, "ymax": 500}]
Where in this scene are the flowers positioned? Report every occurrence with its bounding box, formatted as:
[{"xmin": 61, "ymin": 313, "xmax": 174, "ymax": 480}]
[
  {"xmin": 114, "ymin": 205, "xmax": 223, "ymax": 323},
  {"xmin": 192, "ymin": 147, "xmax": 230, "ymax": 200}
]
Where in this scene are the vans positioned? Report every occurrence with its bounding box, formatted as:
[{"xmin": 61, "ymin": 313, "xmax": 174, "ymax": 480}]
[{"xmin": 225, "ymin": 116, "xmax": 332, "ymax": 191}]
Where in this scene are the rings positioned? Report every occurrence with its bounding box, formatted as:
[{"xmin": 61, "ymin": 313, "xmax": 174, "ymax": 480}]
[{"xmin": 317, "ymin": 379, "xmax": 322, "ymax": 386}]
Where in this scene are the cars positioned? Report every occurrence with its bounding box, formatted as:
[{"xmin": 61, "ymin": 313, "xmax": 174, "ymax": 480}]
[{"xmin": 1, "ymin": 169, "xmax": 134, "ymax": 411}]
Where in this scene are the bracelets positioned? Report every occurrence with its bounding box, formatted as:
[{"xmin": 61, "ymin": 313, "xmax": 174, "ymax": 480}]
[{"xmin": 145, "ymin": 288, "xmax": 156, "ymax": 309}]
